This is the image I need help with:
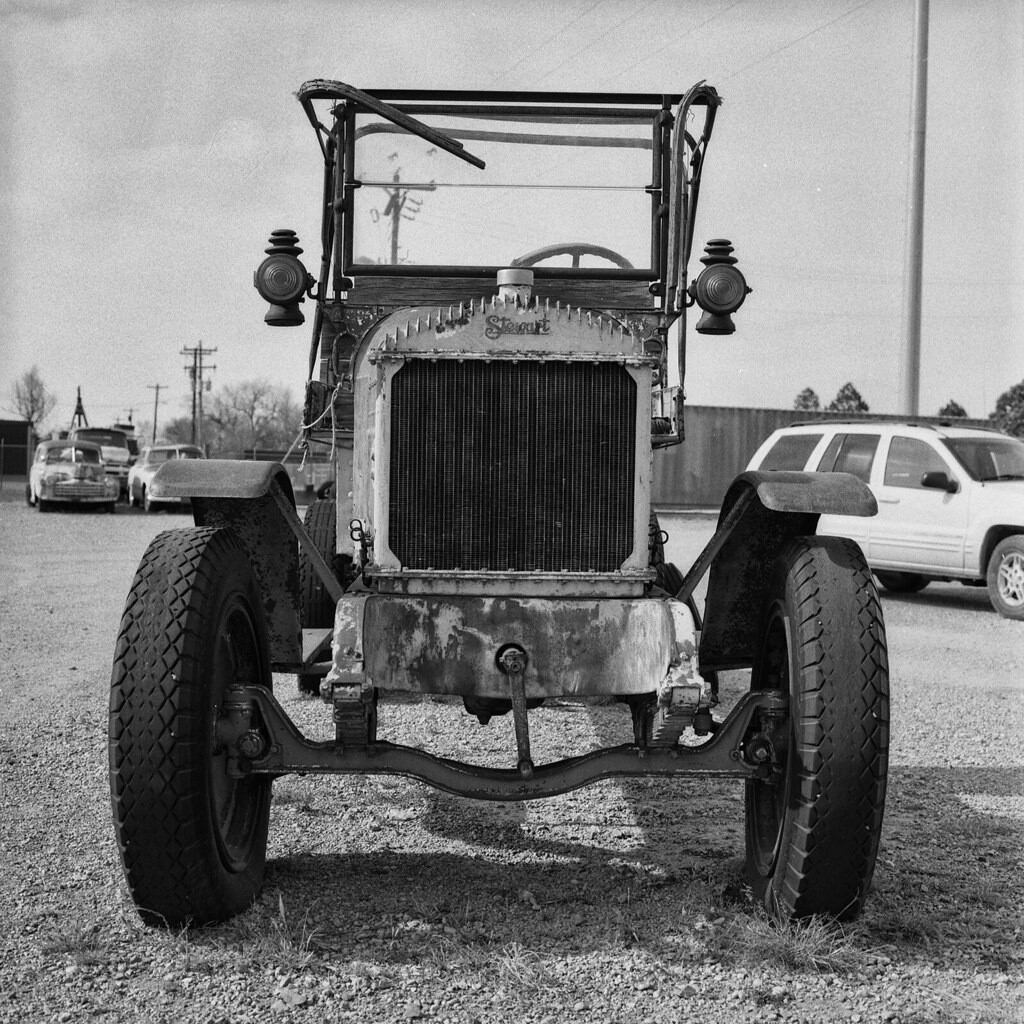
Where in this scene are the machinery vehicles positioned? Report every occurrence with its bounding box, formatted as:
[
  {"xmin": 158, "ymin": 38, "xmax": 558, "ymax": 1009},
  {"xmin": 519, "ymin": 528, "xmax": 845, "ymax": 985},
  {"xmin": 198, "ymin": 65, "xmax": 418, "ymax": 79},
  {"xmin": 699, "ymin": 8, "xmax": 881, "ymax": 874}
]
[{"xmin": 102, "ymin": 73, "xmax": 894, "ymax": 931}]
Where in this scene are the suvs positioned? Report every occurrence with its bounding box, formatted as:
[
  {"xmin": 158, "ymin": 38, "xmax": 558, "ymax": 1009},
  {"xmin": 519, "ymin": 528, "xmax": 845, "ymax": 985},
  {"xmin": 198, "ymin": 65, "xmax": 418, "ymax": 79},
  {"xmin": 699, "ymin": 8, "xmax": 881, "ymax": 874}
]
[{"xmin": 743, "ymin": 417, "xmax": 1024, "ymax": 616}]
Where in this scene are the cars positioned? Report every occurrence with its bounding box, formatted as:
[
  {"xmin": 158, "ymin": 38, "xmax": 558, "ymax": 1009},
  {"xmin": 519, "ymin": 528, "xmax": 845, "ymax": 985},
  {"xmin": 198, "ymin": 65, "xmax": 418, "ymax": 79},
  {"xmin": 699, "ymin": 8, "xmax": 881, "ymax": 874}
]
[
  {"xmin": 127, "ymin": 443, "xmax": 213, "ymax": 513},
  {"xmin": 65, "ymin": 427, "xmax": 140, "ymax": 484},
  {"xmin": 26, "ymin": 439, "xmax": 121, "ymax": 511}
]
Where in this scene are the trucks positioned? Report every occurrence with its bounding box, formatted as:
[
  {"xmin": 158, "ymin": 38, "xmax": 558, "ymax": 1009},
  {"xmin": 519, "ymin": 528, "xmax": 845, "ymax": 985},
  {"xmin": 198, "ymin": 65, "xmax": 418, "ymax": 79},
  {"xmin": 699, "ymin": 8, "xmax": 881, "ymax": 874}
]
[
  {"xmin": 244, "ymin": 448, "xmax": 337, "ymax": 500},
  {"xmin": 109, "ymin": 424, "xmax": 139, "ymax": 456}
]
